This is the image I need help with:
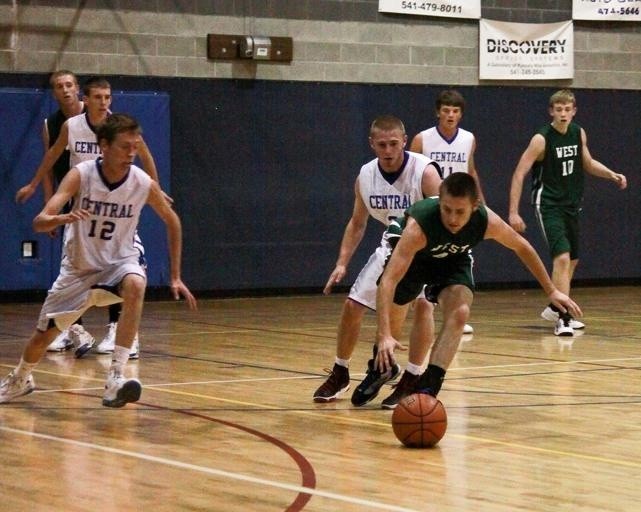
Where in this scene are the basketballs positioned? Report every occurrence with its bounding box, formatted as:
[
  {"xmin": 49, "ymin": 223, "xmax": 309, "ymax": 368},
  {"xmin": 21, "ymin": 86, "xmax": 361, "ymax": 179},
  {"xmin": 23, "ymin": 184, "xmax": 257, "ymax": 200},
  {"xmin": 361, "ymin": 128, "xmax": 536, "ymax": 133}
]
[{"xmin": 392, "ymin": 393, "xmax": 447, "ymax": 447}]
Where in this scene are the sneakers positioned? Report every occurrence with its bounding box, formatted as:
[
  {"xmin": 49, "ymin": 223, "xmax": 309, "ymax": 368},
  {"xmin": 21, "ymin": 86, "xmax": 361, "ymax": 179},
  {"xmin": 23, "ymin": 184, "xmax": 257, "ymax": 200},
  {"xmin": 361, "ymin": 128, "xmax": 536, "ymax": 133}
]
[
  {"xmin": 314, "ymin": 362, "xmax": 350, "ymax": 402},
  {"xmin": 2, "ymin": 324, "xmax": 143, "ymax": 408},
  {"xmin": 352, "ymin": 358, "xmax": 402, "ymax": 407},
  {"xmin": 381, "ymin": 369, "xmax": 420, "ymax": 409},
  {"xmin": 554, "ymin": 312, "xmax": 575, "ymax": 336},
  {"xmin": 409, "ymin": 369, "xmax": 445, "ymax": 397},
  {"xmin": 463, "ymin": 323, "xmax": 474, "ymax": 334},
  {"xmin": 541, "ymin": 303, "xmax": 587, "ymax": 329}
]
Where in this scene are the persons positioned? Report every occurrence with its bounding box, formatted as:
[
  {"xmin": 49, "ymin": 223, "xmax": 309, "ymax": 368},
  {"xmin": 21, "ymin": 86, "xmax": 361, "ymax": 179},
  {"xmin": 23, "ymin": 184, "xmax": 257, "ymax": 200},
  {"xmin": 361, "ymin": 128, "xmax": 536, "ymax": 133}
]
[
  {"xmin": 14, "ymin": 76, "xmax": 174, "ymax": 359},
  {"xmin": 351, "ymin": 174, "xmax": 584, "ymax": 411},
  {"xmin": 409, "ymin": 91, "xmax": 486, "ymax": 333},
  {"xmin": 42, "ymin": 70, "xmax": 122, "ymax": 352},
  {"xmin": 0, "ymin": 112, "xmax": 198, "ymax": 405},
  {"xmin": 313, "ymin": 115, "xmax": 441, "ymax": 412},
  {"xmin": 509, "ymin": 89, "xmax": 626, "ymax": 339}
]
